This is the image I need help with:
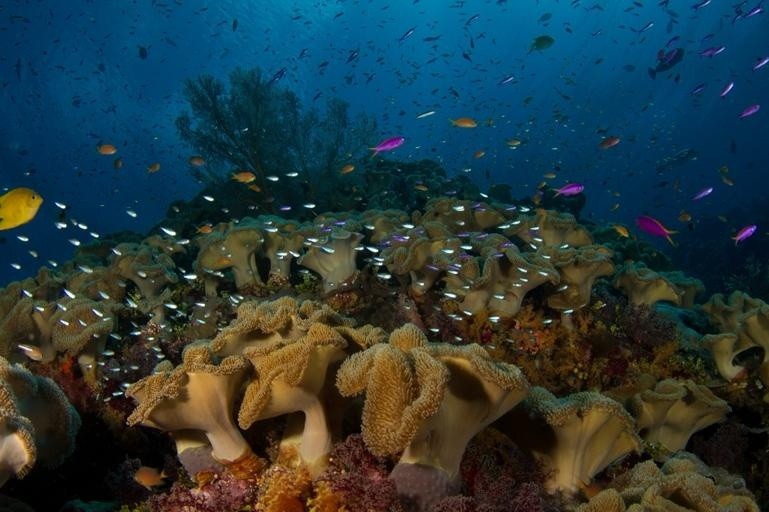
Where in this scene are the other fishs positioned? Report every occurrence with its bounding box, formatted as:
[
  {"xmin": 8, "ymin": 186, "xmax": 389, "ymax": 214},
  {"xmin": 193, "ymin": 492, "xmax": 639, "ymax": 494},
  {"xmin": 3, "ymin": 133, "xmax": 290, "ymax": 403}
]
[{"xmin": 0, "ymin": 0, "xmax": 769, "ymax": 405}]
